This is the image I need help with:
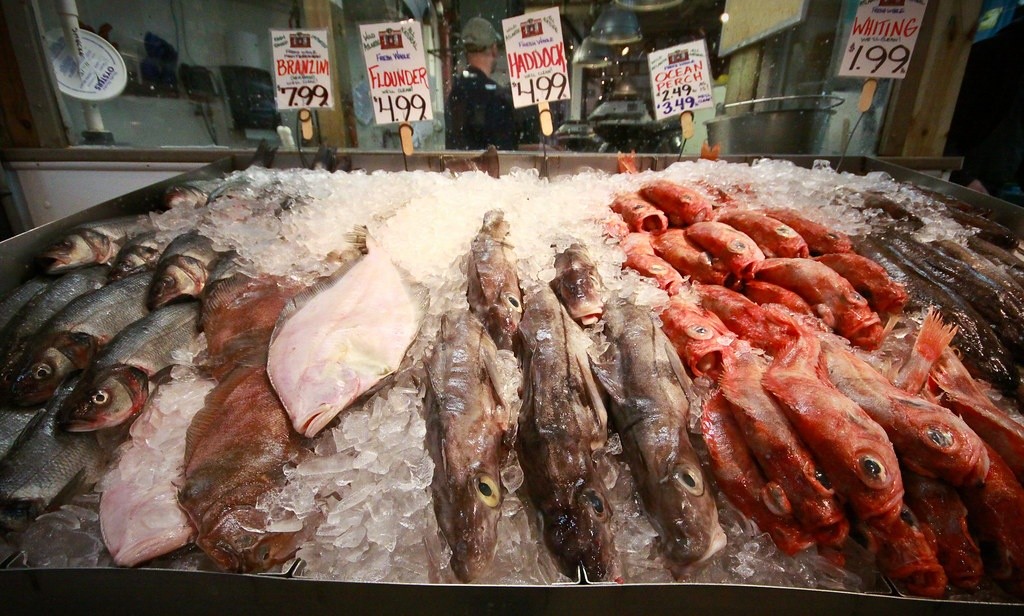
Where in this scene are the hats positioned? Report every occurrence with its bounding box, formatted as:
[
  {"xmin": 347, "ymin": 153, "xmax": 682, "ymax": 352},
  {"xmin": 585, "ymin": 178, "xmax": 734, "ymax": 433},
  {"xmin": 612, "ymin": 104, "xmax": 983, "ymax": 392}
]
[{"xmin": 462, "ymin": 17, "xmax": 502, "ymax": 47}]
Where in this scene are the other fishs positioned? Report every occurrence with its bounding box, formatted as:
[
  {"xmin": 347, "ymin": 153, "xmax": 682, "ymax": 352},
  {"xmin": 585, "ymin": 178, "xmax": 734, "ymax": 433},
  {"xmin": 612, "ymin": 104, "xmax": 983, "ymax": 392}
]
[
  {"xmin": 0, "ymin": 136, "xmax": 433, "ymax": 574},
  {"xmin": 425, "ymin": 139, "xmax": 1024, "ymax": 599}
]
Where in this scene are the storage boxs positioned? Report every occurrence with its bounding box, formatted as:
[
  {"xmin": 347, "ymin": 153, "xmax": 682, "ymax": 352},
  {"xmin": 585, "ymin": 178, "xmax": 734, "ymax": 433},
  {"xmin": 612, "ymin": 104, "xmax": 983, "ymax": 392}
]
[{"xmin": 0, "ymin": 144, "xmax": 1024, "ymax": 616}]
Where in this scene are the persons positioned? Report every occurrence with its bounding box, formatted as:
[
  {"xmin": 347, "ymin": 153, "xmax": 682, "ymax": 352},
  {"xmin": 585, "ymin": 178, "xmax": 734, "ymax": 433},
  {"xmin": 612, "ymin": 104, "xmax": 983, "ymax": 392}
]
[{"xmin": 446, "ymin": 17, "xmax": 520, "ymax": 152}]
